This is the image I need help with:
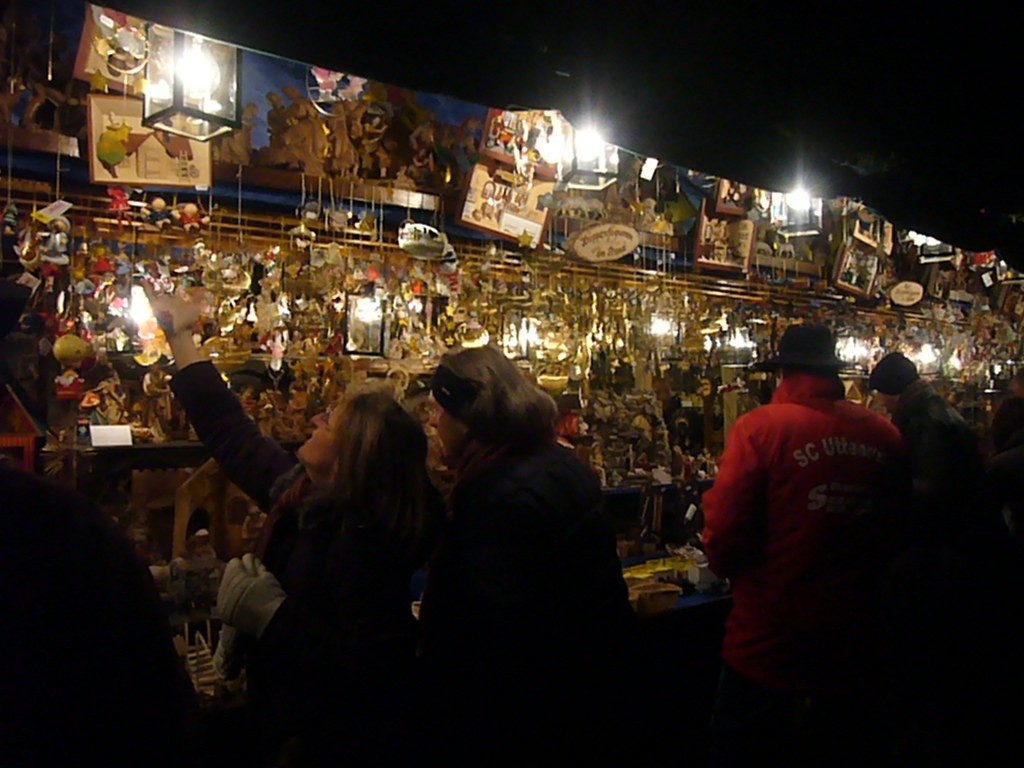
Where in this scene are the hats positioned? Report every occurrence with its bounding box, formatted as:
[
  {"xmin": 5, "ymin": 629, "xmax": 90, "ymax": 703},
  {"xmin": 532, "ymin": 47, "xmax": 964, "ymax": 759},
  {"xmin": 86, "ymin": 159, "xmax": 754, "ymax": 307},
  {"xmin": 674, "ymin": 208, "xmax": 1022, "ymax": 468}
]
[
  {"xmin": 754, "ymin": 325, "xmax": 853, "ymax": 372},
  {"xmin": 869, "ymin": 352, "xmax": 919, "ymax": 394}
]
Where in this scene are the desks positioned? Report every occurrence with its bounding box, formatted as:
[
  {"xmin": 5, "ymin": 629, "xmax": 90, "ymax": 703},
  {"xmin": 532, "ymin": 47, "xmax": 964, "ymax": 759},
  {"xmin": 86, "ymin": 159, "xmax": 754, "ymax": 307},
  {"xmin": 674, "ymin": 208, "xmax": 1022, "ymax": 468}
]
[{"xmin": 608, "ymin": 475, "xmax": 735, "ymax": 632}]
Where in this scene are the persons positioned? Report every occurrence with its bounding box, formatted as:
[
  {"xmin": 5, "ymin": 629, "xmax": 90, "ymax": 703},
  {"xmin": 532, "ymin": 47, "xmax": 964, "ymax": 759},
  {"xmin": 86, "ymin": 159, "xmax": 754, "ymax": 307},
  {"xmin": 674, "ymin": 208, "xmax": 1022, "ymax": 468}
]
[
  {"xmin": 137, "ymin": 274, "xmax": 445, "ymax": 768},
  {"xmin": 869, "ymin": 350, "xmax": 966, "ymax": 481},
  {"xmin": 698, "ymin": 321, "xmax": 903, "ymax": 732},
  {"xmin": 426, "ymin": 347, "xmax": 635, "ymax": 768},
  {"xmin": 981, "ymin": 368, "xmax": 1024, "ymax": 543}
]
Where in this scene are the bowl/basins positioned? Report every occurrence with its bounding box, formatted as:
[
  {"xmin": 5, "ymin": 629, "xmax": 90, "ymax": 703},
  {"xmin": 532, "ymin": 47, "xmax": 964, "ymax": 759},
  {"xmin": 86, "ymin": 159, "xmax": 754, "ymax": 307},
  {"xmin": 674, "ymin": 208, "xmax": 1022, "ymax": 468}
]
[{"xmin": 634, "ymin": 584, "xmax": 681, "ymax": 608}]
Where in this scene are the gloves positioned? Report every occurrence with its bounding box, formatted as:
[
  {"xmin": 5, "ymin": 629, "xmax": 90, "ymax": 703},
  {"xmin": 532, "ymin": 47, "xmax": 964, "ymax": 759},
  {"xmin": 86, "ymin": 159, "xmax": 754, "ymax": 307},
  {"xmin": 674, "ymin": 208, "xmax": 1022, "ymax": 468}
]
[{"xmin": 216, "ymin": 554, "xmax": 286, "ymax": 640}]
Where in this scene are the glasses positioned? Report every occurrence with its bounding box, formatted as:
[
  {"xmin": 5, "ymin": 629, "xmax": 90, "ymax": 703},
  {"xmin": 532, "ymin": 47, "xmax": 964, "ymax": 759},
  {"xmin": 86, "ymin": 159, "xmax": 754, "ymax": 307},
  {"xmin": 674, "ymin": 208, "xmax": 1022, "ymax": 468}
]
[{"xmin": 322, "ymin": 404, "xmax": 337, "ymax": 432}]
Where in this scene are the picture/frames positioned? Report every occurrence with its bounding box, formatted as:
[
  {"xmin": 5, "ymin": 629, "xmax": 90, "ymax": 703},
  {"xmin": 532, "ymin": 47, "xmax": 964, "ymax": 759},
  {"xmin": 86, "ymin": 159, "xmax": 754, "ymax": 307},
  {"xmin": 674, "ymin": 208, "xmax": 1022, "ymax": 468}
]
[
  {"xmin": 713, "ymin": 177, "xmax": 752, "ymax": 218},
  {"xmin": 691, "ymin": 194, "xmax": 758, "ymax": 275},
  {"xmin": 477, "ymin": 107, "xmax": 561, "ymax": 182},
  {"xmin": 84, "ymin": 91, "xmax": 214, "ymax": 195},
  {"xmin": 454, "ymin": 160, "xmax": 563, "ymax": 252},
  {"xmin": 70, "ymin": 4, "xmax": 148, "ymax": 99},
  {"xmin": 834, "ymin": 236, "xmax": 883, "ymax": 301}
]
